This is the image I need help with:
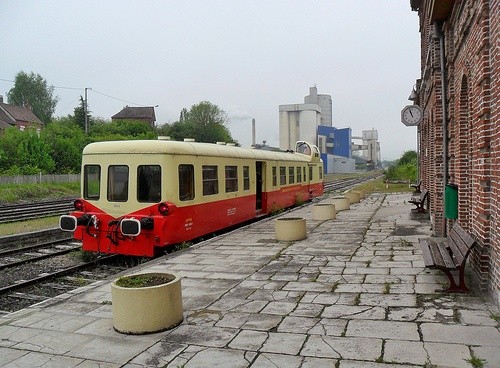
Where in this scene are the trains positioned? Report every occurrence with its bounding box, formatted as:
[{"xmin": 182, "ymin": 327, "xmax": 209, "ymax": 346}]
[{"xmin": 59, "ymin": 134, "xmax": 326, "ymax": 258}]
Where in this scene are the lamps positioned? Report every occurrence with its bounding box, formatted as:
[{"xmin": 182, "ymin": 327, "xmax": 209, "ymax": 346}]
[{"xmin": 408, "ymin": 81, "xmax": 429, "ymax": 100}]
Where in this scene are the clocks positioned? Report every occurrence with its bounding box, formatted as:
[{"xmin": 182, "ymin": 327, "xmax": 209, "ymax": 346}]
[{"xmin": 401, "ymin": 103, "xmax": 423, "ymax": 127}]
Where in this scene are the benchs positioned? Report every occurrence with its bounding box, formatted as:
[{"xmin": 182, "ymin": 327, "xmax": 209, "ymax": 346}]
[
  {"xmin": 419, "ymin": 221, "xmax": 478, "ymax": 293},
  {"xmin": 410, "ymin": 179, "xmax": 423, "ymax": 193},
  {"xmin": 408, "ymin": 188, "xmax": 430, "ymax": 215}
]
